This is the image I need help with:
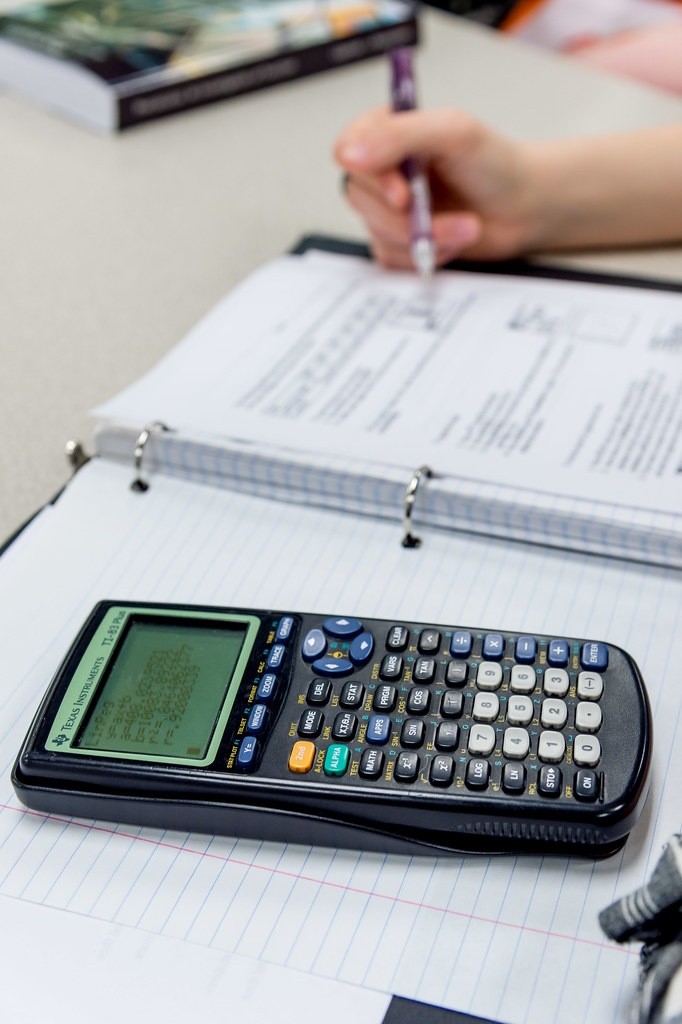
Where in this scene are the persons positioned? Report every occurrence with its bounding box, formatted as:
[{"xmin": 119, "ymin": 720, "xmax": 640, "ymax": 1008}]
[{"xmin": 336, "ymin": 0, "xmax": 682, "ymax": 1023}]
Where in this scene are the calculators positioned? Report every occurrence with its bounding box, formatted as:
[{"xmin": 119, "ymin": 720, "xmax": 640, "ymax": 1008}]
[{"xmin": 10, "ymin": 597, "xmax": 652, "ymax": 863}]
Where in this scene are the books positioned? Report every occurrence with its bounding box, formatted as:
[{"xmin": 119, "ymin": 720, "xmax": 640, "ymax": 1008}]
[
  {"xmin": 0, "ymin": 236, "xmax": 680, "ymax": 1024},
  {"xmin": 0, "ymin": 0, "xmax": 418, "ymax": 131}
]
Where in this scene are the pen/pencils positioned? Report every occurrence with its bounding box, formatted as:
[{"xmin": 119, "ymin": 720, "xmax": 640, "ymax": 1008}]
[{"xmin": 385, "ymin": 45, "xmax": 441, "ymax": 286}]
